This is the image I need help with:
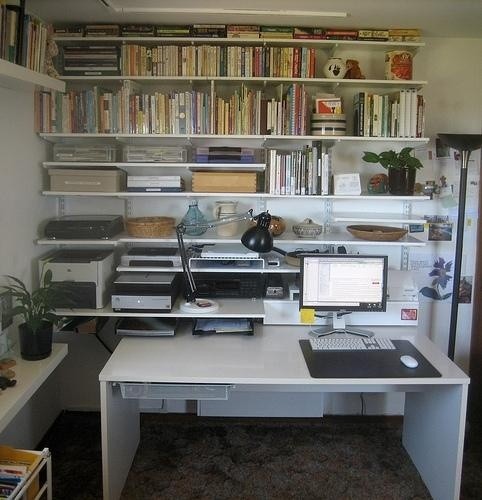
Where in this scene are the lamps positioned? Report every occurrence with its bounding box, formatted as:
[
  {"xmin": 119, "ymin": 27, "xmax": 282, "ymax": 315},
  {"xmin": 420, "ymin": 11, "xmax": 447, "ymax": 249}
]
[
  {"xmin": 174, "ymin": 208, "xmax": 275, "ymax": 314},
  {"xmin": 435, "ymin": 133, "xmax": 481, "ymax": 365}
]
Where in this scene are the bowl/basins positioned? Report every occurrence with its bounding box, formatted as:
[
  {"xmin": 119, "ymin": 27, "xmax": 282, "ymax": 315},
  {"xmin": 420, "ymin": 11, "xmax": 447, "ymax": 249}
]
[
  {"xmin": 311, "ymin": 113, "xmax": 347, "ymax": 137},
  {"xmin": 292, "ymin": 224, "xmax": 322, "ymax": 240},
  {"xmin": 345, "ymin": 225, "xmax": 408, "ymax": 240}
]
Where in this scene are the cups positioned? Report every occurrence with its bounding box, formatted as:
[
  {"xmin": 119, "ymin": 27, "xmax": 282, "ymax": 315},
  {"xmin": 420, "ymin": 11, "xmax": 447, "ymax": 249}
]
[{"xmin": 383, "ymin": 51, "xmax": 413, "ymax": 81}]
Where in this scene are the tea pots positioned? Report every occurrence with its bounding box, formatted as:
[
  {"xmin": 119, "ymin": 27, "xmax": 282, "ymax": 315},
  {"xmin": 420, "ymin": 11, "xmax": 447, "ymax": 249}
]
[{"xmin": 212, "ymin": 200, "xmax": 239, "ymax": 237}]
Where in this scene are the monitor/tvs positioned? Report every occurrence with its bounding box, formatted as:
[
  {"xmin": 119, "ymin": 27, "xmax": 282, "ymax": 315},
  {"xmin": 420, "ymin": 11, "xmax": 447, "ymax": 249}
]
[{"xmin": 301, "ymin": 253, "xmax": 388, "ymax": 337}]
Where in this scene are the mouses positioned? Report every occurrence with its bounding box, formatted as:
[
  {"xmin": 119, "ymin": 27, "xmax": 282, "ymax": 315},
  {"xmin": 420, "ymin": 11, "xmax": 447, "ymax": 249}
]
[{"xmin": 400, "ymin": 355, "xmax": 418, "ymax": 368}]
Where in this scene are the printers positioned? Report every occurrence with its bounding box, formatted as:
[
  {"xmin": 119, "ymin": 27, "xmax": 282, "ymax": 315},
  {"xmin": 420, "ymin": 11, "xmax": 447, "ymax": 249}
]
[{"xmin": 39, "ymin": 249, "xmax": 114, "ymax": 310}]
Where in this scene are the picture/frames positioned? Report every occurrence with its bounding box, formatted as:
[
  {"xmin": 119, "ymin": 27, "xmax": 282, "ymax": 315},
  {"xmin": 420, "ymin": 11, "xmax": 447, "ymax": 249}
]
[{"xmin": 2, "ymin": 288, "xmax": 15, "ymax": 332}]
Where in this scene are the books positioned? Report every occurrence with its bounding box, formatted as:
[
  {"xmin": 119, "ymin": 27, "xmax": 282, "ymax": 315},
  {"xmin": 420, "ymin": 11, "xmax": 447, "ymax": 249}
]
[
  {"xmin": 47, "ymin": 140, "xmax": 362, "ymax": 196},
  {"xmin": 36, "ymin": 26, "xmax": 427, "ymax": 138},
  {"xmin": 116, "ymin": 319, "xmax": 175, "ymax": 337},
  {"xmin": 0, "ymin": 444, "xmax": 43, "ymax": 500},
  {"xmin": 1, "ymin": 5, "xmax": 47, "ymax": 72}
]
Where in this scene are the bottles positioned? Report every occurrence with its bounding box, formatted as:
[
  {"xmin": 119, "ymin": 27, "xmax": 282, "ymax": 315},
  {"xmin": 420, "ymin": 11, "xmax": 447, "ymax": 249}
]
[{"xmin": 181, "ymin": 199, "xmax": 210, "ymax": 236}]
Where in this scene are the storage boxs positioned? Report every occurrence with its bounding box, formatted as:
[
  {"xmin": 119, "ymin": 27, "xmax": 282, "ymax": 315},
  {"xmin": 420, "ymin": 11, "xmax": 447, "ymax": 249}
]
[
  {"xmin": 43, "ymin": 165, "xmax": 126, "ymax": 195},
  {"xmin": 189, "ymin": 169, "xmax": 261, "ymax": 197}
]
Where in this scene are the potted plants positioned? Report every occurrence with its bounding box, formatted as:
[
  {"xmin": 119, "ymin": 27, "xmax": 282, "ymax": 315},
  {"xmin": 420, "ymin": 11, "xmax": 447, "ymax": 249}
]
[
  {"xmin": 360, "ymin": 144, "xmax": 424, "ymax": 195},
  {"xmin": 1, "ymin": 258, "xmax": 82, "ymax": 361}
]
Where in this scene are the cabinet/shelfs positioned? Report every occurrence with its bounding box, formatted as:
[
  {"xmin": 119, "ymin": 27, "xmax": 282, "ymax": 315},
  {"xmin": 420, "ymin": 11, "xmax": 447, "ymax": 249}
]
[{"xmin": 38, "ymin": 33, "xmax": 430, "ymax": 325}]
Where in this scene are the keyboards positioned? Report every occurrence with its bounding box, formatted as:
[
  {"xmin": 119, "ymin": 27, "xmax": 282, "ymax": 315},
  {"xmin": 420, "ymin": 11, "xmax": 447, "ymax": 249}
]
[{"xmin": 309, "ymin": 337, "xmax": 396, "ymax": 351}]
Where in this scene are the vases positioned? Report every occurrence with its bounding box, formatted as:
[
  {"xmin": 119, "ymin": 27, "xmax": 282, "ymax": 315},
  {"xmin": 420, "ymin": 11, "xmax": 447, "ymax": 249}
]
[{"xmin": 309, "ymin": 112, "xmax": 346, "ymax": 136}]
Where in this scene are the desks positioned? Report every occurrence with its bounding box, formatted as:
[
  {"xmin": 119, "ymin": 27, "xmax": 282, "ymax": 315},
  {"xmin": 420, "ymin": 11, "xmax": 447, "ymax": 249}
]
[{"xmin": 97, "ymin": 326, "xmax": 473, "ymax": 499}]
